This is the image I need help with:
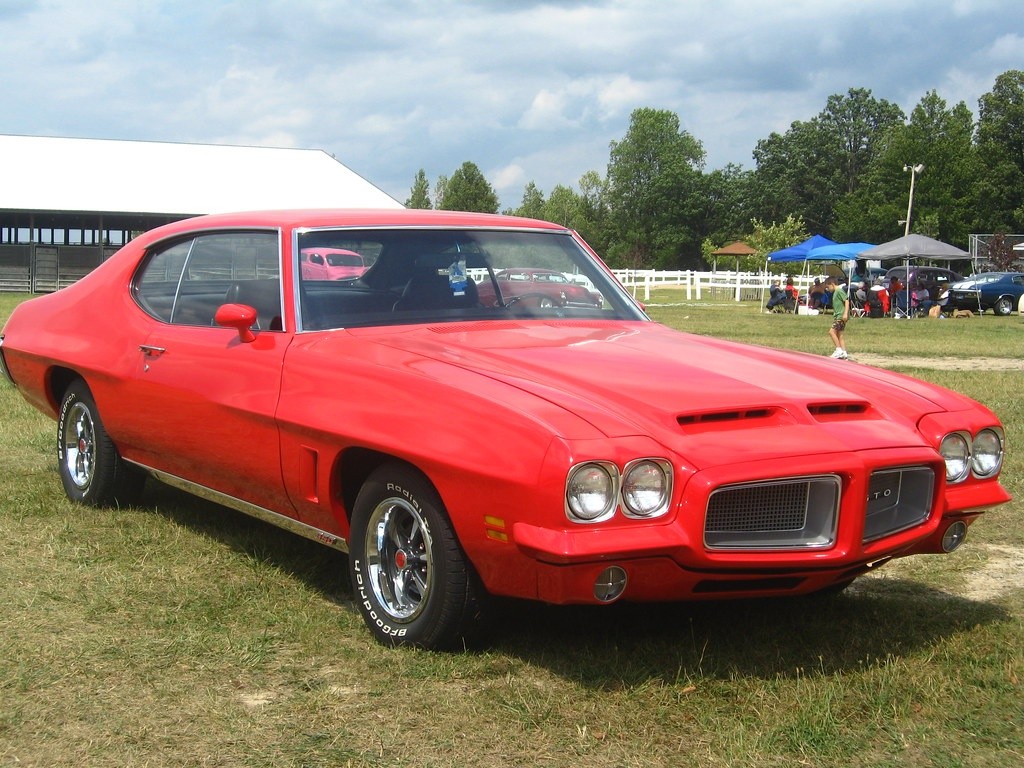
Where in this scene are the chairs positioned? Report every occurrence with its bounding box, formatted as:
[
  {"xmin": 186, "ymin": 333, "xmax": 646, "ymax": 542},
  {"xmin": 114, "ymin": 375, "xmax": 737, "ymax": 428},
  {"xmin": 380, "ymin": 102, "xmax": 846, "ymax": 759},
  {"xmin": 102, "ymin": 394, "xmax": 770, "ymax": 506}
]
[
  {"xmin": 809, "ymin": 292, "xmax": 829, "ymax": 315},
  {"xmin": 865, "ymin": 288, "xmax": 891, "ymax": 318},
  {"xmin": 391, "ymin": 271, "xmax": 480, "ymax": 312},
  {"xmin": 782, "ymin": 289, "xmax": 796, "ymax": 313},
  {"xmin": 848, "ymin": 288, "xmax": 866, "ymax": 318},
  {"xmin": 209, "ymin": 282, "xmax": 321, "ymax": 331},
  {"xmin": 766, "ymin": 288, "xmax": 784, "ymax": 313},
  {"xmin": 933, "ymin": 293, "xmax": 956, "ymax": 318},
  {"xmin": 892, "ymin": 290, "xmax": 912, "ymax": 318}
]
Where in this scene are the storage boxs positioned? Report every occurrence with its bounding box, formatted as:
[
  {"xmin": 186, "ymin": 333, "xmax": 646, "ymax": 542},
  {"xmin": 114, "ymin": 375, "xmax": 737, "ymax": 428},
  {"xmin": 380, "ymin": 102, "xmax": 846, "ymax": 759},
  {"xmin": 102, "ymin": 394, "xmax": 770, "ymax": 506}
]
[{"xmin": 798, "ymin": 306, "xmax": 819, "ymax": 315}]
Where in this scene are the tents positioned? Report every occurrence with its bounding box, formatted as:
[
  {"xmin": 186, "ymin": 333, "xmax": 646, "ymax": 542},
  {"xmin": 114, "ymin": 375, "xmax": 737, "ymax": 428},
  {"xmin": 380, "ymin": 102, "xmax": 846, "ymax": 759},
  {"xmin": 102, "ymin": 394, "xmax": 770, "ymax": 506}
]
[
  {"xmin": 761, "ymin": 231, "xmax": 839, "ymax": 312},
  {"xmin": 857, "ymin": 233, "xmax": 985, "ymax": 317},
  {"xmin": 793, "ymin": 242, "xmax": 877, "ymax": 315}
]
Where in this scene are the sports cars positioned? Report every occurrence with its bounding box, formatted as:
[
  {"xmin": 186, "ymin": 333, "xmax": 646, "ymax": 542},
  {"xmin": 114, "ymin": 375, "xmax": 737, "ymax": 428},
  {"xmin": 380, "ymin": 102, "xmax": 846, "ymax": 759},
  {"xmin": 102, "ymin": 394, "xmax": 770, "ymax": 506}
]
[{"xmin": 2, "ymin": 208, "xmax": 1015, "ymax": 652}]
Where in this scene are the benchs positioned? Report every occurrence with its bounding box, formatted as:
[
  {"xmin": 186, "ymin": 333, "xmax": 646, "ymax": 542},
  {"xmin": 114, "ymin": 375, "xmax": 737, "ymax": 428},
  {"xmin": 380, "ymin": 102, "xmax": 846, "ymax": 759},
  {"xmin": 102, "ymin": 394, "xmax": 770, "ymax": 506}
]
[{"xmin": 146, "ymin": 290, "xmax": 385, "ymax": 326}]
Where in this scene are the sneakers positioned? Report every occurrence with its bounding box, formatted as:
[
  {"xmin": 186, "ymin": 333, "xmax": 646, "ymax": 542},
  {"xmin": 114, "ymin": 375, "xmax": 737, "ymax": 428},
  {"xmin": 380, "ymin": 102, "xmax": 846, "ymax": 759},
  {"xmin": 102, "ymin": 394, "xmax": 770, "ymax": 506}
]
[{"xmin": 830, "ymin": 347, "xmax": 847, "ymax": 360}]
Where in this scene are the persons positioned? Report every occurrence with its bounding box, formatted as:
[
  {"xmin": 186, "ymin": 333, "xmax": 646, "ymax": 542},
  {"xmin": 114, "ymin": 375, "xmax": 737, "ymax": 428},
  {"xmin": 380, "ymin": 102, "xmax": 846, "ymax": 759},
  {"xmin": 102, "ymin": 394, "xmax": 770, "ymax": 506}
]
[
  {"xmin": 820, "ymin": 275, "xmax": 851, "ymax": 360},
  {"xmin": 769, "ymin": 270, "xmax": 933, "ymax": 317}
]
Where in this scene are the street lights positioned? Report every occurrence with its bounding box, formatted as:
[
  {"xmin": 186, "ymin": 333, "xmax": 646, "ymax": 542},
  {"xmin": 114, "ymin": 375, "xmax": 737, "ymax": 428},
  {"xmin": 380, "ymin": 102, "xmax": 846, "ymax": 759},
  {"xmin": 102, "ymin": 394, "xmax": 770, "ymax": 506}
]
[{"xmin": 902, "ymin": 163, "xmax": 925, "ymax": 265}]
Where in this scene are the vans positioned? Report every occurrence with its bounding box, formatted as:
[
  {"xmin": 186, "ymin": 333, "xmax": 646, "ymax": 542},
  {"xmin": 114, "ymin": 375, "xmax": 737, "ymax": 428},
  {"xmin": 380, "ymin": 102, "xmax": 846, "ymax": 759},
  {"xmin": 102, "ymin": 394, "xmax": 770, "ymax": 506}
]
[{"xmin": 881, "ymin": 266, "xmax": 967, "ymax": 301}]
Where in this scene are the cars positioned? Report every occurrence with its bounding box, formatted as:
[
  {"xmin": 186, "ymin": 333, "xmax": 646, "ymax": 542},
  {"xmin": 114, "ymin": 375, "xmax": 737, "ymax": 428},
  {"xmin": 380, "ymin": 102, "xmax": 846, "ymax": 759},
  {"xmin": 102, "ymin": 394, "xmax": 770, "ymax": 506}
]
[
  {"xmin": 838, "ymin": 267, "xmax": 888, "ymax": 284},
  {"xmin": 938, "ymin": 270, "xmax": 1024, "ymax": 315},
  {"xmin": 300, "ymin": 247, "xmax": 366, "ymax": 283},
  {"xmin": 475, "ymin": 267, "xmax": 603, "ymax": 309}
]
